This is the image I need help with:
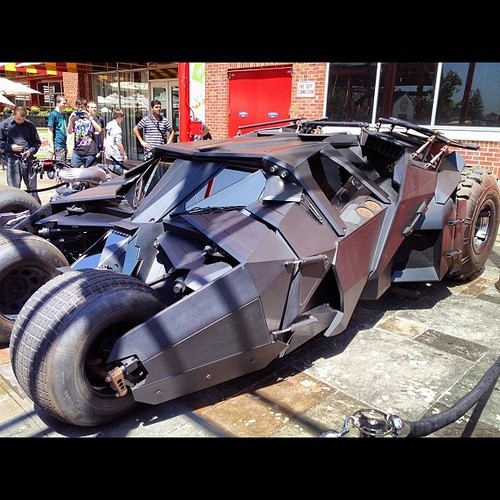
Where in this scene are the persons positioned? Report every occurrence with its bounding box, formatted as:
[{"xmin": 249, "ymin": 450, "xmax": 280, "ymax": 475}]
[
  {"xmin": 133, "ymin": 99, "xmax": 175, "ymax": 160},
  {"xmin": 69, "ymin": 99, "xmax": 128, "ymax": 167},
  {"xmin": 48, "ymin": 95, "xmax": 67, "ymax": 189},
  {"xmin": 0, "ymin": 106, "xmax": 41, "ymax": 204}
]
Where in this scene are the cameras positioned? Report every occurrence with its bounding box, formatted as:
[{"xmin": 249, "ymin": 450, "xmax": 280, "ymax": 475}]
[{"xmin": 75, "ymin": 112, "xmax": 85, "ymax": 118}]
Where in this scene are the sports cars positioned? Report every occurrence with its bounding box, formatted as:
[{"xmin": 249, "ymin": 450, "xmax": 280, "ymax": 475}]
[{"xmin": 0, "ymin": 116, "xmax": 500, "ymax": 427}]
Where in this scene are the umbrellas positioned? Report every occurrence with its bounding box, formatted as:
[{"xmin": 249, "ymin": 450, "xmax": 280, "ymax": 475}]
[{"xmin": 0, "ymin": 77, "xmax": 41, "ymax": 106}]
